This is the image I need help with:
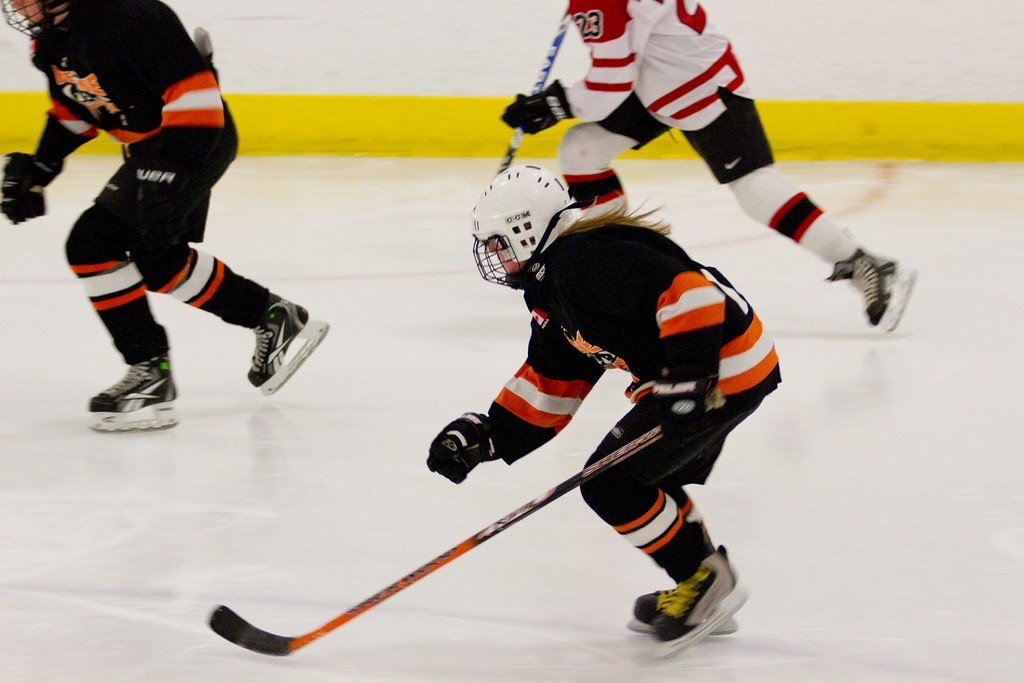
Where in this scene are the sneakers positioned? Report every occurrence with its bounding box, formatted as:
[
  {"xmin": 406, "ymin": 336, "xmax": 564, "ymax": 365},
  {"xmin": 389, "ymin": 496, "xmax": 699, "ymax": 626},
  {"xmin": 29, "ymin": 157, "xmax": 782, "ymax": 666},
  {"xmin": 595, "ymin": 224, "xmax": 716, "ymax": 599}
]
[
  {"xmin": 650, "ymin": 545, "xmax": 748, "ymax": 659},
  {"xmin": 627, "ymin": 584, "xmax": 740, "ymax": 639},
  {"xmin": 248, "ymin": 294, "xmax": 330, "ymax": 396},
  {"xmin": 828, "ymin": 247, "xmax": 919, "ymax": 333},
  {"xmin": 88, "ymin": 356, "xmax": 179, "ymax": 432}
]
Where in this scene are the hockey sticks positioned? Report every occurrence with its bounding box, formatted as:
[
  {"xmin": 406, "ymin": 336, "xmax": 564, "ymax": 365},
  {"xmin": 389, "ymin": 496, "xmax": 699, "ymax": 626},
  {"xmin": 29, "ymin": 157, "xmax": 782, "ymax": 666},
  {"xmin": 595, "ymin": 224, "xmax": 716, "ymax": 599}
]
[
  {"xmin": 194, "ymin": 27, "xmax": 213, "ymax": 64},
  {"xmin": 497, "ymin": 5, "xmax": 573, "ymax": 173},
  {"xmin": 205, "ymin": 388, "xmax": 726, "ymax": 657}
]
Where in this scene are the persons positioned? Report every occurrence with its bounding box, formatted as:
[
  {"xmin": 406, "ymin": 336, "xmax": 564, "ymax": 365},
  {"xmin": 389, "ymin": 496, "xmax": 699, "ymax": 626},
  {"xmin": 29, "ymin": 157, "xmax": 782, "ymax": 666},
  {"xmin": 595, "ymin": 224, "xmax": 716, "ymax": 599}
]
[
  {"xmin": 0, "ymin": 0, "xmax": 329, "ymax": 430},
  {"xmin": 501, "ymin": 0, "xmax": 914, "ymax": 332},
  {"xmin": 427, "ymin": 166, "xmax": 782, "ymax": 660}
]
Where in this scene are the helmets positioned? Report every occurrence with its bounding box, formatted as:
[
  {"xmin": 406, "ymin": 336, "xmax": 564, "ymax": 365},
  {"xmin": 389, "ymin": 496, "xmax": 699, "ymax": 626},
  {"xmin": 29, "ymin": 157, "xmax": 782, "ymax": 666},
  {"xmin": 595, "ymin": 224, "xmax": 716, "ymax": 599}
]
[{"xmin": 470, "ymin": 164, "xmax": 583, "ymax": 263}]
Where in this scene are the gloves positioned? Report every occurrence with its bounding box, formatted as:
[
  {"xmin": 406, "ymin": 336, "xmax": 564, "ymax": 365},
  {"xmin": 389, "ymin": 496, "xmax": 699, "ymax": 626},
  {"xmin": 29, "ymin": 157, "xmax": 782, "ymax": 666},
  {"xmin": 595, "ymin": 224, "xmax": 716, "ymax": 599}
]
[
  {"xmin": 647, "ymin": 374, "xmax": 714, "ymax": 451},
  {"xmin": 501, "ymin": 78, "xmax": 575, "ymax": 135},
  {"xmin": 0, "ymin": 150, "xmax": 64, "ymax": 225},
  {"xmin": 426, "ymin": 411, "xmax": 499, "ymax": 484}
]
